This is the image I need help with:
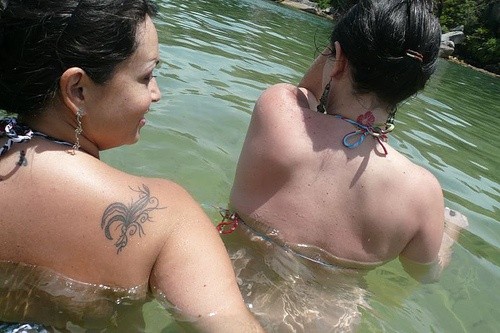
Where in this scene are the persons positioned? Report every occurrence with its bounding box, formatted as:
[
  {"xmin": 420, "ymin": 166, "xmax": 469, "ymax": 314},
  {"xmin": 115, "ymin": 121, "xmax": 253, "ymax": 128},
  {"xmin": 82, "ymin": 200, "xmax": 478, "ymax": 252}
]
[
  {"xmin": 223, "ymin": 0, "xmax": 469, "ymax": 333},
  {"xmin": 0, "ymin": 0, "xmax": 268, "ymax": 333}
]
